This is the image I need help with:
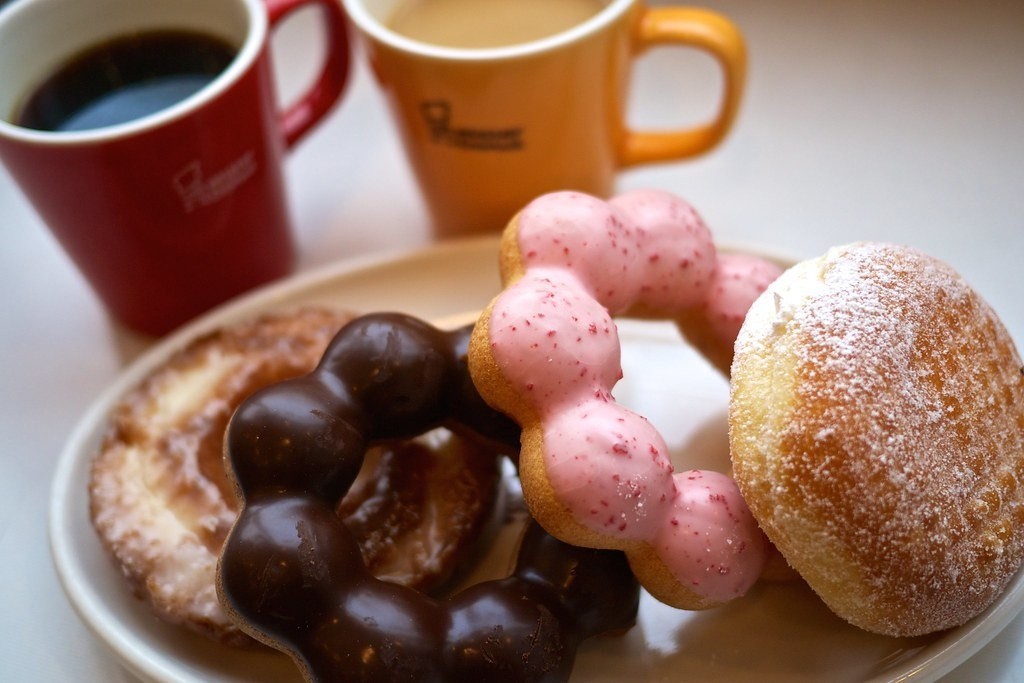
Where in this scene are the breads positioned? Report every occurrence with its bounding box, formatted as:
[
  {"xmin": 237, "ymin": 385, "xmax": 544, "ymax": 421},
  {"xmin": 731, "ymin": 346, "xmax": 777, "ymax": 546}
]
[{"xmin": 728, "ymin": 239, "xmax": 1024, "ymax": 637}]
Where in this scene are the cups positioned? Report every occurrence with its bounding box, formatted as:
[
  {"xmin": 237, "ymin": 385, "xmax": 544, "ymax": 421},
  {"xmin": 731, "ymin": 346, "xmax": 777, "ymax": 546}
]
[
  {"xmin": 0, "ymin": 0, "xmax": 354, "ymax": 345},
  {"xmin": 339, "ymin": 0, "xmax": 749, "ymax": 243}
]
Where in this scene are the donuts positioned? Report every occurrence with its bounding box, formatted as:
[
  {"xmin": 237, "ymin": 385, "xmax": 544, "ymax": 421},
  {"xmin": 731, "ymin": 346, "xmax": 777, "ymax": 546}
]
[
  {"xmin": 220, "ymin": 310, "xmax": 638, "ymax": 683},
  {"xmin": 89, "ymin": 313, "xmax": 498, "ymax": 647},
  {"xmin": 467, "ymin": 191, "xmax": 782, "ymax": 609}
]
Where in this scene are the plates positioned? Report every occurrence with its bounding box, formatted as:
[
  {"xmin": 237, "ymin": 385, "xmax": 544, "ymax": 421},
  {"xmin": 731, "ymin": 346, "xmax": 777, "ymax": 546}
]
[{"xmin": 48, "ymin": 239, "xmax": 1024, "ymax": 683}]
